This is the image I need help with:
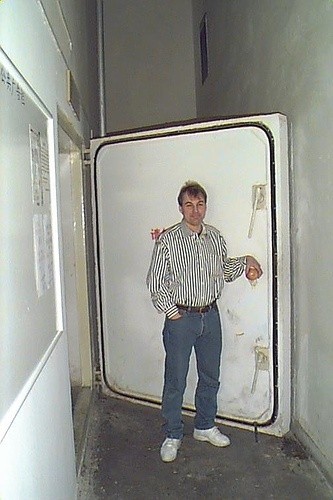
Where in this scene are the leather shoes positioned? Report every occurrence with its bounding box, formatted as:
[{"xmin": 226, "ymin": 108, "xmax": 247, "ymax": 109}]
[{"xmin": 176, "ymin": 299, "xmax": 215, "ymax": 314}]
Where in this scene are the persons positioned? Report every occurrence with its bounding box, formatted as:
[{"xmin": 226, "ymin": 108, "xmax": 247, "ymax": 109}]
[{"xmin": 145, "ymin": 180, "xmax": 263, "ymax": 463}]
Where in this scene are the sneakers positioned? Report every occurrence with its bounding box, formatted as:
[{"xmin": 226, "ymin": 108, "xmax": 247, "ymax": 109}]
[
  {"xmin": 193, "ymin": 426, "xmax": 229, "ymax": 447},
  {"xmin": 160, "ymin": 437, "xmax": 180, "ymax": 462}
]
